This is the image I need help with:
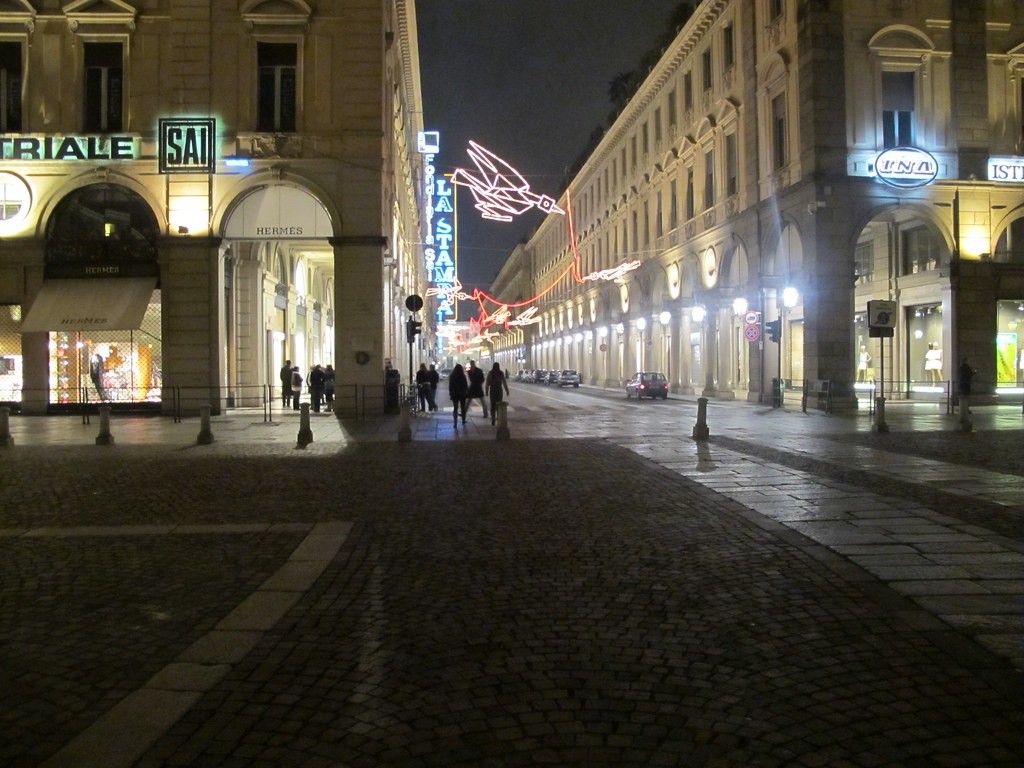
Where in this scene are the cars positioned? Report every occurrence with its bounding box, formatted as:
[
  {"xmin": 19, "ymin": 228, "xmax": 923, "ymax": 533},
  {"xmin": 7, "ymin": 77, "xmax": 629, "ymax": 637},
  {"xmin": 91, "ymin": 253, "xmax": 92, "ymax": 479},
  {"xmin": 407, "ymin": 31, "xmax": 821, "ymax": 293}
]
[
  {"xmin": 438, "ymin": 369, "xmax": 451, "ymax": 382},
  {"xmin": 557, "ymin": 370, "xmax": 580, "ymax": 388},
  {"xmin": 513, "ymin": 369, "xmax": 562, "ymax": 386},
  {"xmin": 626, "ymin": 372, "xmax": 669, "ymax": 400}
]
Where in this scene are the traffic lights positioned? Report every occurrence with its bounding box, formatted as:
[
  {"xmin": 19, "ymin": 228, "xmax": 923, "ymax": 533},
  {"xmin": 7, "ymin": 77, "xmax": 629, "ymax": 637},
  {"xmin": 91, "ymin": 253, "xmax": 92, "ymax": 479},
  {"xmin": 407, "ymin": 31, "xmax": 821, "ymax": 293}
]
[
  {"xmin": 766, "ymin": 322, "xmax": 781, "ymax": 343},
  {"xmin": 411, "ymin": 322, "xmax": 422, "ymax": 336}
]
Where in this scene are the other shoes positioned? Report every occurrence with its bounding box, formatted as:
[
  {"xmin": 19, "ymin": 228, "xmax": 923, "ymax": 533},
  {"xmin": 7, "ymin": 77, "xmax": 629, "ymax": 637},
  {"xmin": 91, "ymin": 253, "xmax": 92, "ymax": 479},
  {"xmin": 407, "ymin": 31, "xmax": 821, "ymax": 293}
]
[
  {"xmin": 324, "ymin": 409, "xmax": 331, "ymax": 412},
  {"xmin": 483, "ymin": 413, "xmax": 489, "ymax": 418},
  {"xmin": 463, "ymin": 421, "xmax": 466, "ymax": 426},
  {"xmin": 458, "ymin": 414, "xmax": 465, "ymax": 417},
  {"xmin": 454, "ymin": 425, "xmax": 457, "ymax": 430},
  {"xmin": 293, "ymin": 408, "xmax": 301, "ymax": 410},
  {"xmin": 491, "ymin": 423, "xmax": 495, "ymax": 426}
]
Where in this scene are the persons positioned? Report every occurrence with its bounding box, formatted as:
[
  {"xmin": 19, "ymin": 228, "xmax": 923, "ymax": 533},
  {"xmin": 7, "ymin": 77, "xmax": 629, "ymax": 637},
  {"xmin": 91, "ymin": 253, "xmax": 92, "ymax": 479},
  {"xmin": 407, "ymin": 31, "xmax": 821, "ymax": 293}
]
[
  {"xmin": 416, "ymin": 363, "xmax": 438, "ymax": 412},
  {"xmin": 457, "ymin": 360, "xmax": 488, "ymax": 418},
  {"xmin": 429, "ymin": 364, "xmax": 440, "ymax": 411},
  {"xmin": 486, "ymin": 362, "xmax": 510, "ymax": 426},
  {"xmin": 925, "ymin": 341, "xmax": 943, "ymax": 387},
  {"xmin": 857, "ymin": 345, "xmax": 871, "ymax": 381},
  {"xmin": 448, "ymin": 364, "xmax": 468, "ymax": 429},
  {"xmin": 280, "ymin": 360, "xmax": 336, "ymax": 412}
]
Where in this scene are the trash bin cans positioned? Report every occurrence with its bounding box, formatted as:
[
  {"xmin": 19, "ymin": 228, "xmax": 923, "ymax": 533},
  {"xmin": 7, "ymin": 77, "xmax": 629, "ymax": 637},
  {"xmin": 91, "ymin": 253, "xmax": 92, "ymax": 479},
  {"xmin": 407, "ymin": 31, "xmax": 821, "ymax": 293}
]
[
  {"xmin": 773, "ymin": 379, "xmax": 784, "ymax": 405},
  {"xmin": 385, "ymin": 369, "xmax": 401, "ymax": 413}
]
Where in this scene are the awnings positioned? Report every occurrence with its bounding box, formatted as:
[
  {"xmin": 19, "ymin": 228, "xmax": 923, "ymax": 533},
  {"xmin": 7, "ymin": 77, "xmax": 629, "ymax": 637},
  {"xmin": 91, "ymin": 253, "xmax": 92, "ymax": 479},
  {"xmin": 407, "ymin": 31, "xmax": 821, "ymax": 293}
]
[{"xmin": 18, "ymin": 277, "xmax": 164, "ymax": 346}]
